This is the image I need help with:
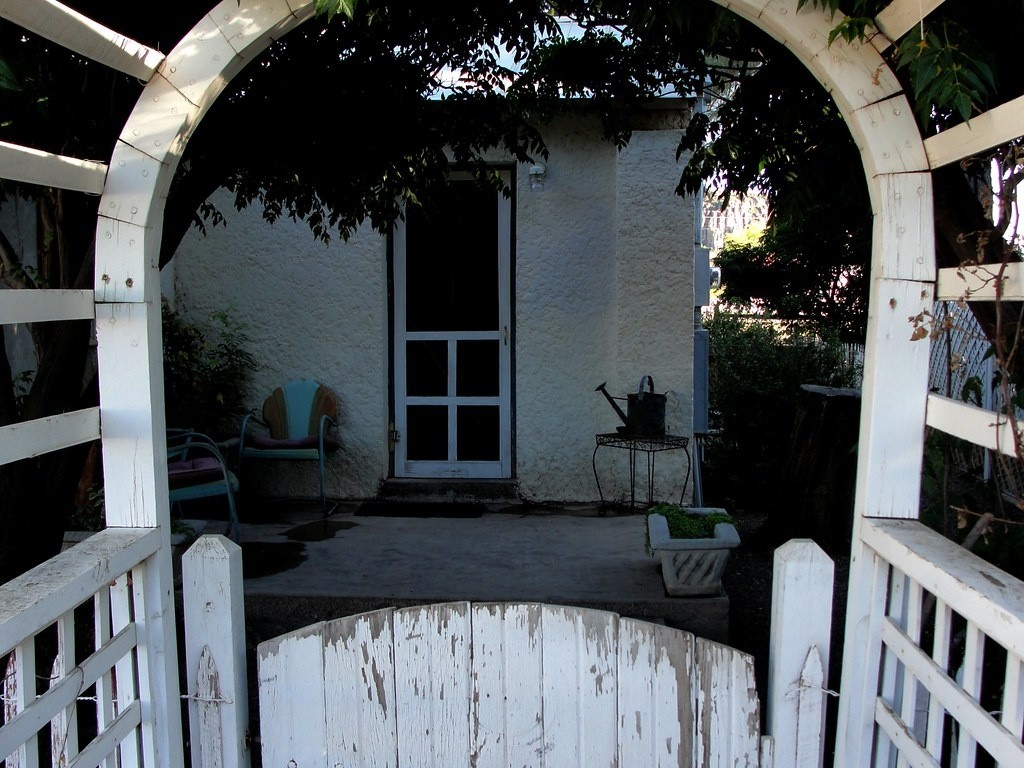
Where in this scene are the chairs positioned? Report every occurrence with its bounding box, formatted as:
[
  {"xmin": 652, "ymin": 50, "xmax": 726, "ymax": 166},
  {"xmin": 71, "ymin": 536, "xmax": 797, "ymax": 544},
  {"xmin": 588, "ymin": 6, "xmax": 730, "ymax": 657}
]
[
  {"xmin": 237, "ymin": 377, "xmax": 342, "ymax": 518},
  {"xmin": 169, "ymin": 432, "xmax": 240, "ymax": 546}
]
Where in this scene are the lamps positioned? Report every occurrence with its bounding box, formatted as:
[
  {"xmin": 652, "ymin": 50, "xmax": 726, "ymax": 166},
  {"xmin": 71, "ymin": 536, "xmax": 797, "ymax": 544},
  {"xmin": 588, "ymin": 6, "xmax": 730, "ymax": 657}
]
[{"xmin": 529, "ymin": 161, "xmax": 546, "ymax": 191}]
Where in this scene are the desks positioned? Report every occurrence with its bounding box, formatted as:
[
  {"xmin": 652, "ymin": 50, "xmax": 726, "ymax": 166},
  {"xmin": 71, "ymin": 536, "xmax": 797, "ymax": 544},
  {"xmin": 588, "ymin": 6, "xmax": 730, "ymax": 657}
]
[{"xmin": 593, "ymin": 433, "xmax": 692, "ymax": 516}]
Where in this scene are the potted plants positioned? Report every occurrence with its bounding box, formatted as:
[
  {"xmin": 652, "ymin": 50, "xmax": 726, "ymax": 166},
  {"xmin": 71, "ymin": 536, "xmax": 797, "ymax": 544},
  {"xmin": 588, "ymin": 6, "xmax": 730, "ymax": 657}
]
[
  {"xmin": 171, "ymin": 518, "xmax": 208, "ymax": 590},
  {"xmin": 643, "ymin": 503, "xmax": 742, "ymax": 597},
  {"xmin": 161, "ymin": 305, "xmax": 254, "ymax": 470}
]
